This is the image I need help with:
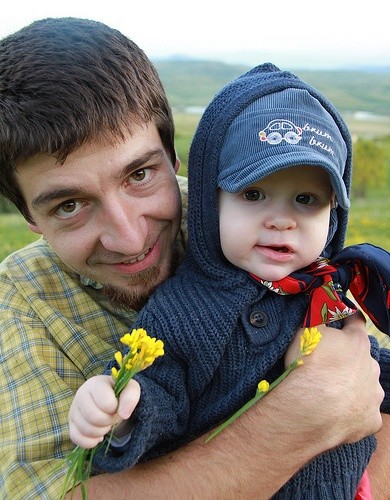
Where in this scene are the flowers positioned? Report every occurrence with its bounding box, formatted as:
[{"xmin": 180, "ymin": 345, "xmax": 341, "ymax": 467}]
[
  {"xmin": 24, "ymin": 328, "xmax": 164, "ymax": 500},
  {"xmin": 204, "ymin": 327, "xmax": 322, "ymax": 444}
]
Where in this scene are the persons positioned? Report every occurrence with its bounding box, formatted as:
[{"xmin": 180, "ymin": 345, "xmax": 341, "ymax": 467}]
[
  {"xmin": 66, "ymin": 61, "xmax": 390, "ymax": 499},
  {"xmin": 0, "ymin": 16, "xmax": 390, "ymax": 500}
]
